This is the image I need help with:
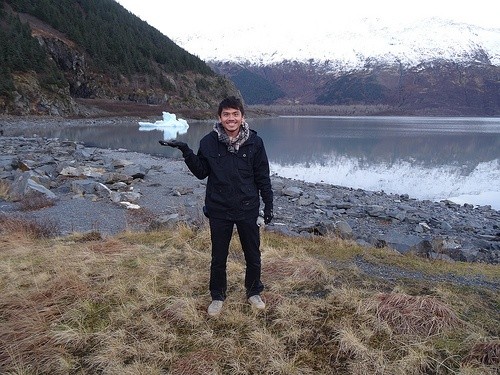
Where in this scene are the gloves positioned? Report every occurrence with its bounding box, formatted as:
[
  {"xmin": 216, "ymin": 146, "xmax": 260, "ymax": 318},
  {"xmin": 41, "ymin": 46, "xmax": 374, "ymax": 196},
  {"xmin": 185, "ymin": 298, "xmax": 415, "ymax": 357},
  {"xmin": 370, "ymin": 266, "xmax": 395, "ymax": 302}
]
[
  {"xmin": 264, "ymin": 204, "xmax": 274, "ymax": 224},
  {"xmin": 158, "ymin": 140, "xmax": 187, "ymax": 149}
]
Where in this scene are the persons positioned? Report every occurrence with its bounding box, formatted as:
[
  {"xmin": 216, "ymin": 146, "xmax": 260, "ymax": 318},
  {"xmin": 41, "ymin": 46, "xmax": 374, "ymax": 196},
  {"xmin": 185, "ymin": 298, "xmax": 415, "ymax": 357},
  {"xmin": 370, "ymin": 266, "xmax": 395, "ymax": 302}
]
[{"xmin": 158, "ymin": 95, "xmax": 274, "ymax": 317}]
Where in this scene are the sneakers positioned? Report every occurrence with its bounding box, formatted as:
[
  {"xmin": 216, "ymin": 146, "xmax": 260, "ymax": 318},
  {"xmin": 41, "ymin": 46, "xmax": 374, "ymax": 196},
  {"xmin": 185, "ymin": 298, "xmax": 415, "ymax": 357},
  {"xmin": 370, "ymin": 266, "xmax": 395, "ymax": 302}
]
[
  {"xmin": 248, "ymin": 296, "xmax": 265, "ymax": 309},
  {"xmin": 208, "ymin": 300, "xmax": 223, "ymax": 316}
]
